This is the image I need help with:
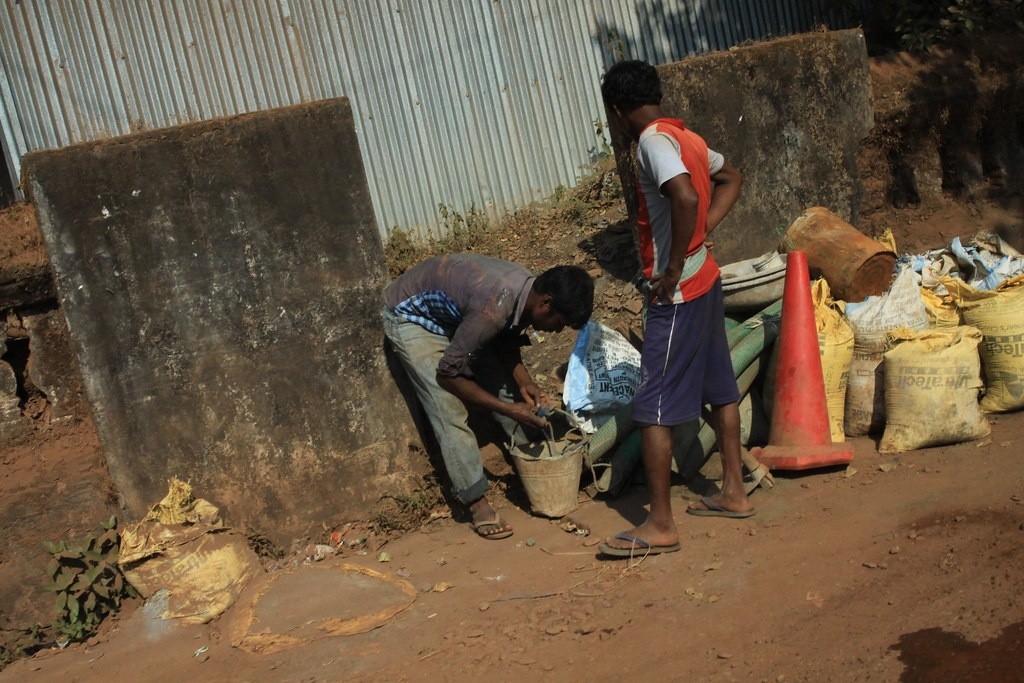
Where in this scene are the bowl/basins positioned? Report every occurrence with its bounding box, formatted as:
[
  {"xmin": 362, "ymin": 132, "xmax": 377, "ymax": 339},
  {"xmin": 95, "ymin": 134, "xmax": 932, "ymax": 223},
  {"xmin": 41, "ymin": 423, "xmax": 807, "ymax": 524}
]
[{"xmin": 718, "ymin": 253, "xmax": 787, "ymax": 311}]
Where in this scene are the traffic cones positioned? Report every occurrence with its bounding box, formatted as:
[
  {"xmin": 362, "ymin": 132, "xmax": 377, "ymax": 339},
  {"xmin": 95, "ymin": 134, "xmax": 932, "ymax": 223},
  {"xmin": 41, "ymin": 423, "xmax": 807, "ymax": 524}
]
[{"xmin": 745, "ymin": 248, "xmax": 853, "ymax": 472}]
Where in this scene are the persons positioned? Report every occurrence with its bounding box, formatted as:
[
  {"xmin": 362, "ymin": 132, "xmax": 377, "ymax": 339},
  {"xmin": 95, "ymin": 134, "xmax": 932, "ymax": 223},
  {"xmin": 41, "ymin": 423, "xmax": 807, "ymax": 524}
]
[
  {"xmin": 381, "ymin": 252, "xmax": 594, "ymax": 540},
  {"xmin": 597, "ymin": 59, "xmax": 756, "ymax": 556}
]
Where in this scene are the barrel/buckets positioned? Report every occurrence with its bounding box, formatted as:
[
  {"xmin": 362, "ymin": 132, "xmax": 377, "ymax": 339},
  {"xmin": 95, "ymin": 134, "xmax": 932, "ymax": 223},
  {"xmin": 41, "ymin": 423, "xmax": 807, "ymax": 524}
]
[
  {"xmin": 752, "ymin": 248, "xmax": 784, "ymax": 272},
  {"xmin": 778, "ymin": 206, "xmax": 899, "ymax": 303},
  {"xmin": 503, "ymin": 407, "xmax": 590, "ymax": 518}
]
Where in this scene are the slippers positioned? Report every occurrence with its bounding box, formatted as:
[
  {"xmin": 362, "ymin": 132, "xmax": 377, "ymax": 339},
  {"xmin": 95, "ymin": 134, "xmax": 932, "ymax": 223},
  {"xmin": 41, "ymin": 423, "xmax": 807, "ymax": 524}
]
[
  {"xmin": 687, "ymin": 496, "xmax": 759, "ymax": 518},
  {"xmin": 598, "ymin": 528, "xmax": 682, "ymax": 555},
  {"xmin": 470, "ymin": 502, "xmax": 514, "ymax": 540}
]
